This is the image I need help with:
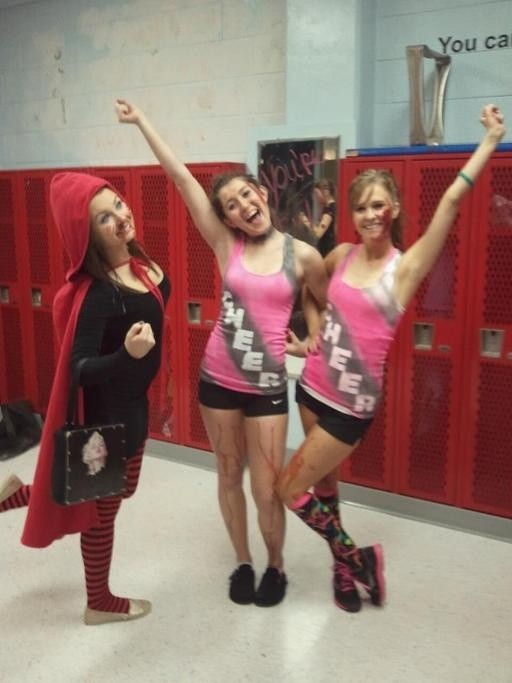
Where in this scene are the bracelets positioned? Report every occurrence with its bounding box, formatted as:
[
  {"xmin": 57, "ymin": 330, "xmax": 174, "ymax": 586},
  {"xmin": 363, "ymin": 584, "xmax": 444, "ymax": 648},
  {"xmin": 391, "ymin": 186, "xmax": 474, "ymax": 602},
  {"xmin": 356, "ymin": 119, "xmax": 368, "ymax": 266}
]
[{"xmin": 457, "ymin": 170, "xmax": 475, "ymax": 188}]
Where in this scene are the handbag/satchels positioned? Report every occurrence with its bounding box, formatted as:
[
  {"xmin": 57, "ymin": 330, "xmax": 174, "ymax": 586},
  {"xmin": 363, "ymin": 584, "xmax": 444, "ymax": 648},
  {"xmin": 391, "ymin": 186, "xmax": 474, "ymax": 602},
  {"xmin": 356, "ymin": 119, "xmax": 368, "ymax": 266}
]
[
  {"xmin": 51, "ymin": 359, "xmax": 127, "ymax": 507},
  {"xmin": 0, "ymin": 399, "xmax": 44, "ymax": 459}
]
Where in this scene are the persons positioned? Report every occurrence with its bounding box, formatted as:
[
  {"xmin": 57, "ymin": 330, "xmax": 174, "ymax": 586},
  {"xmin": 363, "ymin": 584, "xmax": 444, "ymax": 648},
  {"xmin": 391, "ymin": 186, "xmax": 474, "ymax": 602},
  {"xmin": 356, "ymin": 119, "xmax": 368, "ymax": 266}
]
[
  {"xmin": 21, "ymin": 170, "xmax": 175, "ymax": 628},
  {"xmin": 269, "ymin": 97, "xmax": 506, "ymax": 611},
  {"xmin": 293, "ymin": 178, "xmax": 337, "ymax": 257},
  {"xmin": 111, "ymin": 94, "xmax": 333, "ymax": 608}
]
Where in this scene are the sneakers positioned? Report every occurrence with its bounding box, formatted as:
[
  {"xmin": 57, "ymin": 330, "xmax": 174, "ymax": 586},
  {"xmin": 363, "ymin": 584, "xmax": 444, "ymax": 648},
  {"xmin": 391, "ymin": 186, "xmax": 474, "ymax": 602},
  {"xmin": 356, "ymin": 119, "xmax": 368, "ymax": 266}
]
[
  {"xmin": 347, "ymin": 543, "xmax": 385, "ymax": 605},
  {"xmin": 255, "ymin": 567, "xmax": 287, "ymax": 606},
  {"xmin": 333, "ymin": 563, "xmax": 360, "ymax": 612},
  {"xmin": 229, "ymin": 563, "xmax": 255, "ymax": 605}
]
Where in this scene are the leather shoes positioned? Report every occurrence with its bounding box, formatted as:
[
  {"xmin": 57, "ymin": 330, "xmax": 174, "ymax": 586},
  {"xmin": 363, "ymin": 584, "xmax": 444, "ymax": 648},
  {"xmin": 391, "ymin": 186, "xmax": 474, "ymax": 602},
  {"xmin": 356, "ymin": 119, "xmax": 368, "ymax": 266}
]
[
  {"xmin": 84, "ymin": 598, "xmax": 151, "ymax": 625},
  {"xmin": 0, "ymin": 473, "xmax": 23, "ymax": 502}
]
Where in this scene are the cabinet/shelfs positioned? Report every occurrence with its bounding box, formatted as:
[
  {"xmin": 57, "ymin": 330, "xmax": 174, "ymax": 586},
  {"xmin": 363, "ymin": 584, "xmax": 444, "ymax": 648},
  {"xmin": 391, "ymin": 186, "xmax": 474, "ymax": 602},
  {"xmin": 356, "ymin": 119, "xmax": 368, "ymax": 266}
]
[
  {"xmin": 334, "ymin": 152, "xmax": 512, "ymax": 521},
  {"xmin": 0, "ymin": 161, "xmax": 251, "ymax": 452}
]
